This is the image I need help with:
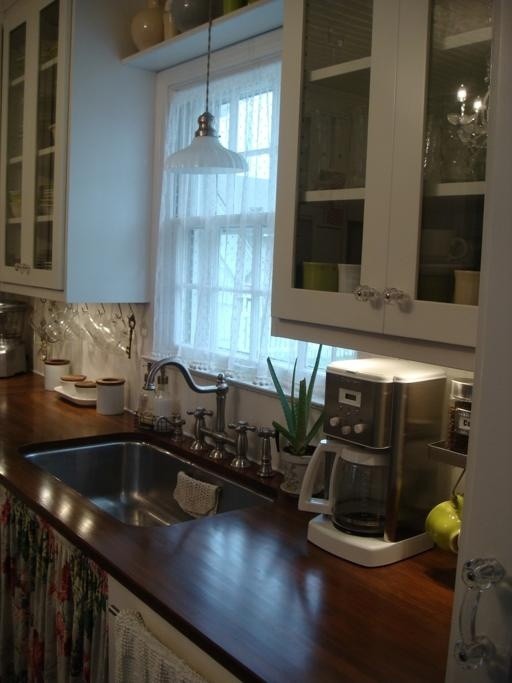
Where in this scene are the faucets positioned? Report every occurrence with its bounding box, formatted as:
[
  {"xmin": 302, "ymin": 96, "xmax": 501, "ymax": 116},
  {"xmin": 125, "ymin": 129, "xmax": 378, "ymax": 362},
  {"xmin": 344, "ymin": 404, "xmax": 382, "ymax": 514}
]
[{"xmin": 143, "ymin": 357, "xmax": 235, "ymax": 459}]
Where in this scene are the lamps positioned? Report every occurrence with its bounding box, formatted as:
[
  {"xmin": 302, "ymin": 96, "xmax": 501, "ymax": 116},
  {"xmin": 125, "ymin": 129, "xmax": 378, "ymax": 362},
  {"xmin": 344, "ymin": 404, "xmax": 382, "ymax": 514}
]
[
  {"xmin": 166, "ymin": 0, "xmax": 249, "ymax": 173},
  {"xmin": 443, "ymin": 55, "xmax": 490, "ymax": 159}
]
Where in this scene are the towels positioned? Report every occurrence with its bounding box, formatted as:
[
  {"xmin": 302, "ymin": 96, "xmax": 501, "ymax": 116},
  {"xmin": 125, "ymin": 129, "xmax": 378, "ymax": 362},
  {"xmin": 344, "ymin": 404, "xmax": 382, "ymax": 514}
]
[
  {"xmin": 172, "ymin": 471, "xmax": 219, "ymax": 520},
  {"xmin": 112, "ymin": 608, "xmax": 207, "ymax": 682}
]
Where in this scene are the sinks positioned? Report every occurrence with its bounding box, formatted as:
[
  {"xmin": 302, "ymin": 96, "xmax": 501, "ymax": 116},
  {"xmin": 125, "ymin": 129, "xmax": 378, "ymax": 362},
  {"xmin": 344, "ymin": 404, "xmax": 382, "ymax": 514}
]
[{"xmin": 16, "ymin": 432, "xmax": 275, "ymax": 528}]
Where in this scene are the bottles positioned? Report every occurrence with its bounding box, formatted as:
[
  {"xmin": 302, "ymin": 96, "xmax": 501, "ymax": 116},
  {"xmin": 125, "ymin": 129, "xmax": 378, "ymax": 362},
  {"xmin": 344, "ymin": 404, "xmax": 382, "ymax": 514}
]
[
  {"xmin": 137, "ymin": 362, "xmax": 156, "ymax": 420},
  {"xmin": 150, "ymin": 374, "xmax": 175, "ymax": 428}
]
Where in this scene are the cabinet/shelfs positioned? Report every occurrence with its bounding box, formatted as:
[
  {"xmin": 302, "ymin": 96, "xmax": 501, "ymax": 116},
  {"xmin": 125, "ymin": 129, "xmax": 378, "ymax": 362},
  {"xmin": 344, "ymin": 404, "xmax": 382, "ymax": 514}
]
[
  {"xmin": 1, "ymin": 0, "xmax": 158, "ymax": 306},
  {"xmin": 270, "ymin": 2, "xmax": 512, "ymax": 371}
]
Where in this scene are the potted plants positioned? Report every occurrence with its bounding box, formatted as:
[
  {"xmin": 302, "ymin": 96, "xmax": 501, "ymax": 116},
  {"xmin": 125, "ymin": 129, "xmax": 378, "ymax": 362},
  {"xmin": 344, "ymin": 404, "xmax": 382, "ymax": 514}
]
[{"xmin": 268, "ymin": 341, "xmax": 326, "ymax": 495}]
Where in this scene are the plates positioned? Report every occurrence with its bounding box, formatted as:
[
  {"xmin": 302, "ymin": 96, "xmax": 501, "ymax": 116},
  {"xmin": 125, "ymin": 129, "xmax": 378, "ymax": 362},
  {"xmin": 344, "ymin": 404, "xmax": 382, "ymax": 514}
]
[{"xmin": 8, "ymin": 116, "xmax": 55, "ymax": 274}]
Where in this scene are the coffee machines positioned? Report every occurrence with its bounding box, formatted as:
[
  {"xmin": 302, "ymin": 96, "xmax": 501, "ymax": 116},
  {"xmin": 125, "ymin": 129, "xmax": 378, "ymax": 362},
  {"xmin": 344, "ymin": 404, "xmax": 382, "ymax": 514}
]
[{"xmin": 295, "ymin": 356, "xmax": 447, "ymax": 570}]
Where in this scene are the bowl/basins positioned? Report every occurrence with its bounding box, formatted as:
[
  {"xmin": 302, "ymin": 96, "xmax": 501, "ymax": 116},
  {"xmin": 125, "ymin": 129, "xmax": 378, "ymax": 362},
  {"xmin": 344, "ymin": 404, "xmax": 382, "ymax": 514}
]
[{"xmin": 424, "ymin": 494, "xmax": 461, "ymax": 549}]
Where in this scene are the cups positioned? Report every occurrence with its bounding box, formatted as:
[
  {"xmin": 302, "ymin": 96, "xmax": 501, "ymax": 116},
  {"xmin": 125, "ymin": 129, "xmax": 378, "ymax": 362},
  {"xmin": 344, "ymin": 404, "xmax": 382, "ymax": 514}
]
[
  {"xmin": 300, "ymin": 261, "xmax": 327, "ymax": 293},
  {"xmin": 453, "ymin": 270, "xmax": 479, "ymax": 307},
  {"xmin": 419, "ymin": 228, "xmax": 468, "ymax": 262},
  {"xmin": 43, "ymin": 303, "xmax": 134, "ymax": 354},
  {"xmin": 337, "ymin": 259, "xmax": 361, "ymax": 297}
]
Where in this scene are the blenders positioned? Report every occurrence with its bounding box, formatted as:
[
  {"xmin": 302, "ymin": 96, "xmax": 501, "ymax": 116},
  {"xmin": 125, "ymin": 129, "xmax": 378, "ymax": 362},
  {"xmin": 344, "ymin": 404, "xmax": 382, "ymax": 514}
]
[{"xmin": 1, "ymin": 300, "xmax": 31, "ymax": 379}]
